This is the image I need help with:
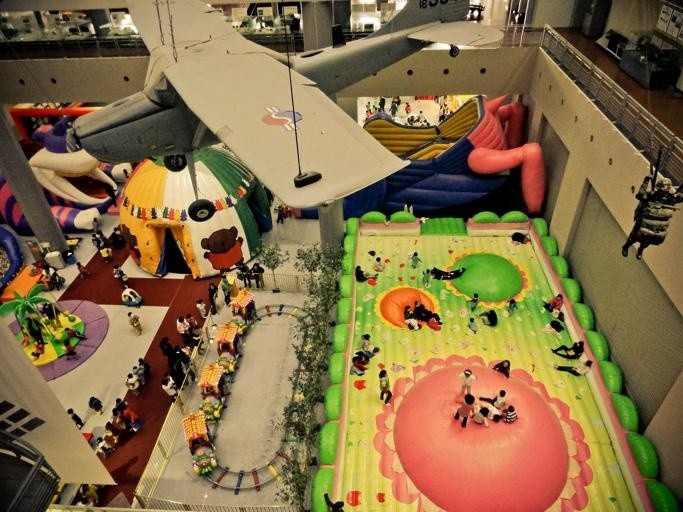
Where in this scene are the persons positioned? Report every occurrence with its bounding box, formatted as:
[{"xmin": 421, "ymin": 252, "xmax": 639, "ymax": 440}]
[
  {"xmin": 620, "ymin": 174, "xmax": 683, "ymax": 260},
  {"xmin": 360, "ymin": 93, "xmax": 453, "ymax": 128},
  {"xmin": 22, "ymin": 201, "xmax": 293, "ymax": 506},
  {"xmin": 319, "ymin": 486, "xmax": 345, "ymax": 511},
  {"xmin": 348, "ymin": 232, "xmax": 595, "ymax": 429}
]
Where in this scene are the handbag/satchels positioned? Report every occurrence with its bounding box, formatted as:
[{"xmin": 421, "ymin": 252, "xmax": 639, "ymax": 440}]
[
  {"xmin": 134, "ymin": 321, "xmax": 140, "ymax": 327},
  {"xmin": 32, "ymin": 321, "xmax": 39, "ymax": 331},
  {"xmin": 122, "ymin": 274, "xmax": 128, "ymax": 281}
]
